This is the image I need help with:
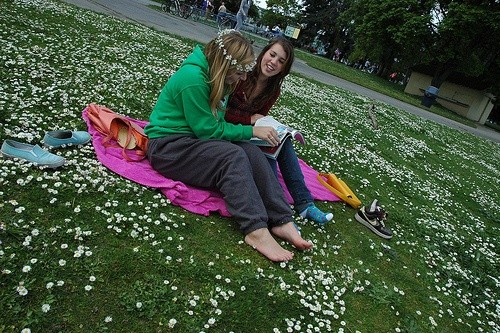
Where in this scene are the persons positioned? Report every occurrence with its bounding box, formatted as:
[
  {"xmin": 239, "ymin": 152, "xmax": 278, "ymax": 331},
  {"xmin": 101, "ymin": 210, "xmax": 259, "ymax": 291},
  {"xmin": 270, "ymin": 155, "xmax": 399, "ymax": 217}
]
[
  {"xmin": 334, "ymin": 48, "xmax": 341, "ymax": 60},
  {"xmin": 201, "ymin": 0, "xmax": 208, "ymax": 17},
  {"xmin": 145, "ymin": 30, "xmax": 312, "ymax": 261},
  {"xmin": 235, "ymin": 0, "xmax": 251, "ymax": 31},
  {"xmin": 225, "ymin": 35, "xmax": 334, "ymax": 236},
  {"xmin": 216, "ymin": 2, "xmax": 227, "ymax": 15},
  {"xmin": 364, "ymin": 59, "xmax": 370, "ymax": 68},
  {"xmin": 371, "ymin": 62, "xmax": 377, "ymax": 72},
  {"xmin": 271, "ymin": 23, "xmax": 281, "ymax": 32}
]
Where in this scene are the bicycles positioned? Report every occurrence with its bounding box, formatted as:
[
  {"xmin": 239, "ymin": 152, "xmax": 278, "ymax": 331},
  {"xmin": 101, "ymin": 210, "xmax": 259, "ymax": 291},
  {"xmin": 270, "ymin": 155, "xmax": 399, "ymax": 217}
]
[{"xmin": 161, "ymin": 0, "xmax": 202, "ymax": 22}]
